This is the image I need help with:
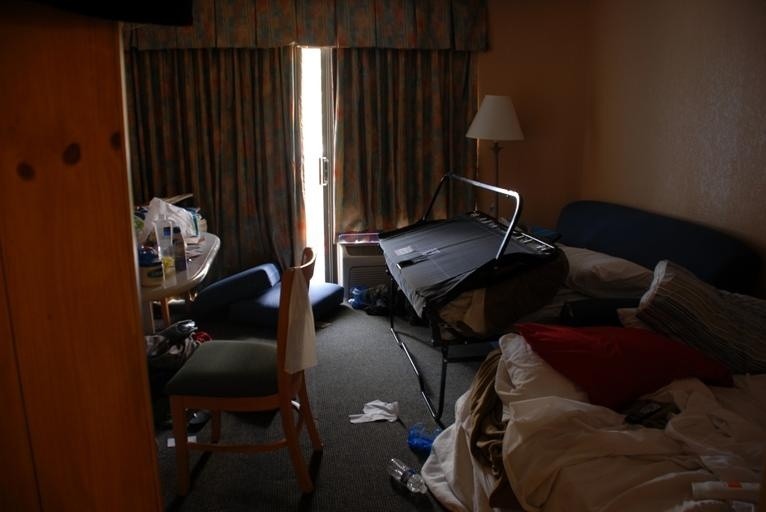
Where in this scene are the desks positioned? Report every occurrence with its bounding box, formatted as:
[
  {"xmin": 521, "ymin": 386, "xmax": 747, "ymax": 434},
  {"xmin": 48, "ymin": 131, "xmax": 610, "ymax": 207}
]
[{"xmin": 139, "ymin": 232, "xmax": 222, "ymax": 338}]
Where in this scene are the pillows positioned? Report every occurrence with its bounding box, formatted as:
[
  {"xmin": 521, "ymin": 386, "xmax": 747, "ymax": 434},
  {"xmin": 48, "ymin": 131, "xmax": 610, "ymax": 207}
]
[
  {"xmin": 616, "ymin": 307, "xmax": 649, "ymax": 330},
  {"xmin": 636, "ymin": 257, "xmax": 766, "ymax": 375},
  {"xmin": 511, "ymin": 320, "xmax": 737, "ymax": 408}
]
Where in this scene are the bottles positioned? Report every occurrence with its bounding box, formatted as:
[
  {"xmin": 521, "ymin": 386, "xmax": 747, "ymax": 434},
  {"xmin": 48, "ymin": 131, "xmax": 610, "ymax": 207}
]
[
  {"xmin": 385, "ymin": 458, "xmax": 427, "ymax": 496},
  {"xmin": 161, "ymin": 226, "xmax": 187, "ymax": 272}
]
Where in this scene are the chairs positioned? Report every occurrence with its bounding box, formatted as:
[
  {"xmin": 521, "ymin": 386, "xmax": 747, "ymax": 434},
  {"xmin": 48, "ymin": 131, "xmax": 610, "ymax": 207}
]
[{"xmin": 166, "ymin": 248, "xmax": 323, "ymax": 495}]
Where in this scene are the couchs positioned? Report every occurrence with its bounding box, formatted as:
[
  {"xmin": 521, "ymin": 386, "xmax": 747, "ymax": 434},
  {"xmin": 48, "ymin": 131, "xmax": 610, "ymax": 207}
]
[{"xmin": 554, "ymin": 199, "xmax": 763, "ymax": 343}]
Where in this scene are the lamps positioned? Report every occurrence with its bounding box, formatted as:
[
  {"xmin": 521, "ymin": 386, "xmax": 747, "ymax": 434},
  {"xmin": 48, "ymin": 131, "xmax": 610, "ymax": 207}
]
[{"xmin": 465, "ymin": 94, "xmax": 525, "ymax": 220}]
